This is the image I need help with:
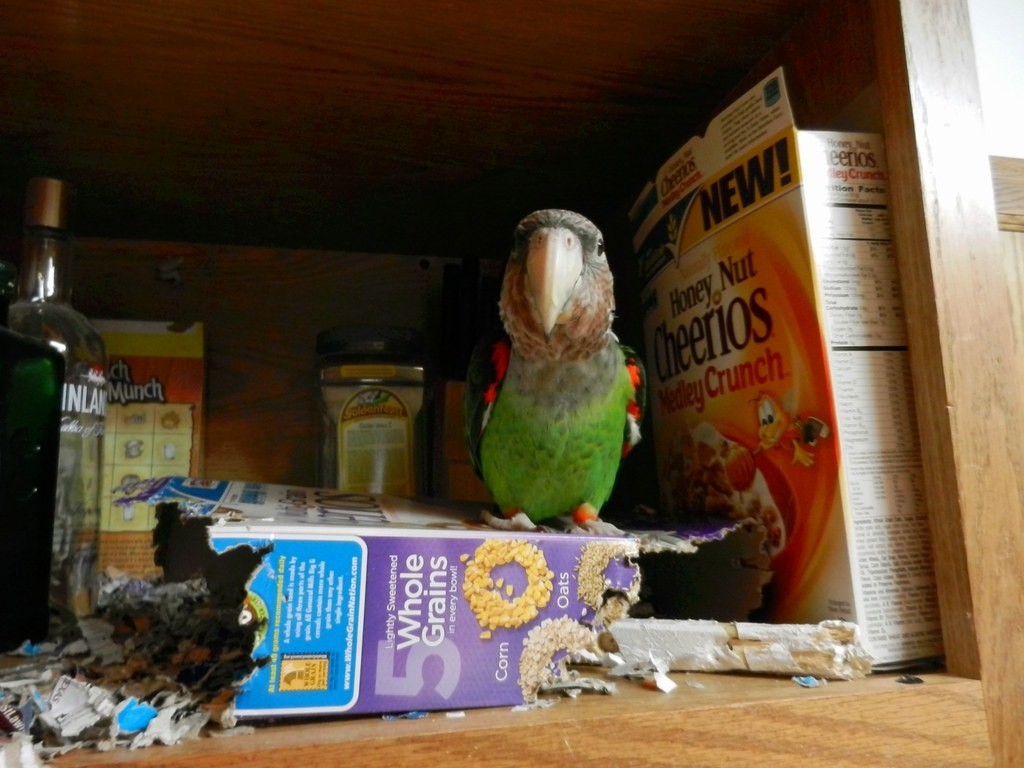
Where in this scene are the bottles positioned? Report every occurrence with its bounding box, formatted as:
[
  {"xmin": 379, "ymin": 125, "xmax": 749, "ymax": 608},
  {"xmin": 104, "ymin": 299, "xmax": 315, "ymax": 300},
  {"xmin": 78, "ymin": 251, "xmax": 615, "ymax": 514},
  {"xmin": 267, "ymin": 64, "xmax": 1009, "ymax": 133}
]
[
  {"xmin": 8, "ymin": 179, "xmax": 108, "ymax": 636},
  {"xmin": 0, "ymin": 260, "xmax": 65, "ymax": 649}
]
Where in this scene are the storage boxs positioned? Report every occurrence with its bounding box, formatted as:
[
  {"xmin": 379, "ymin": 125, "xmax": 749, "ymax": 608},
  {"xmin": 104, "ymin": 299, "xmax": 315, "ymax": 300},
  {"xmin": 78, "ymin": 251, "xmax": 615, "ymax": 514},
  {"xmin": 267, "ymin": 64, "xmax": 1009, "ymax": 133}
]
[
  {"xmin": 628, "ymin": 67, "xmax": 949, "ymax": 673},
  {"xmin": 84, "ymin": 317, "xmax": 207, "ymax": 586},
  {"xmin": 103, "ymin": 479, "xmax": 757, "ymax": 732}
]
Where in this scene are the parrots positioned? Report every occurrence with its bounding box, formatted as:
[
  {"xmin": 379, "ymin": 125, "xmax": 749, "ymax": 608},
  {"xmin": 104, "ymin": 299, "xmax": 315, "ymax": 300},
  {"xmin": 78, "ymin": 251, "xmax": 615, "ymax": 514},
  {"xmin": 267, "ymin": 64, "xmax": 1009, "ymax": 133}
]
[{"xmin": 461, "ymin": 209, "xmax": 648, "ymax": 538}]
